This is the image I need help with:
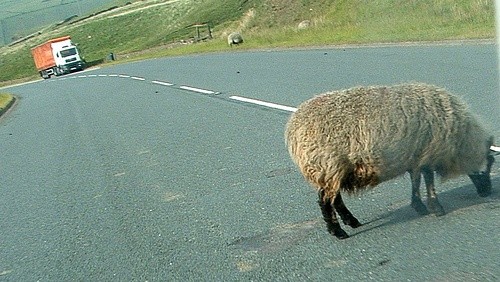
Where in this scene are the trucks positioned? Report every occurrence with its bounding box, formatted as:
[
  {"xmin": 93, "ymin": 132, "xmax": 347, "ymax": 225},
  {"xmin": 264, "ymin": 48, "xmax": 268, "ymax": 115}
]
[{"xmin": 30, "ymin": 35, "xmax": 84, "ymax": 79}]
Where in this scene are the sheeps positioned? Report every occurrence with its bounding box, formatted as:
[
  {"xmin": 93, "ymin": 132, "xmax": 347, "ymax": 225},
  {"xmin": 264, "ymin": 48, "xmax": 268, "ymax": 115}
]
[
  {"xmin": 284, "ymin": 82, "xmax": 500, "ymax": 240},
  {"xmin": 228, "ymin": 32, "xmax": 243, "ymax": 48}
]
